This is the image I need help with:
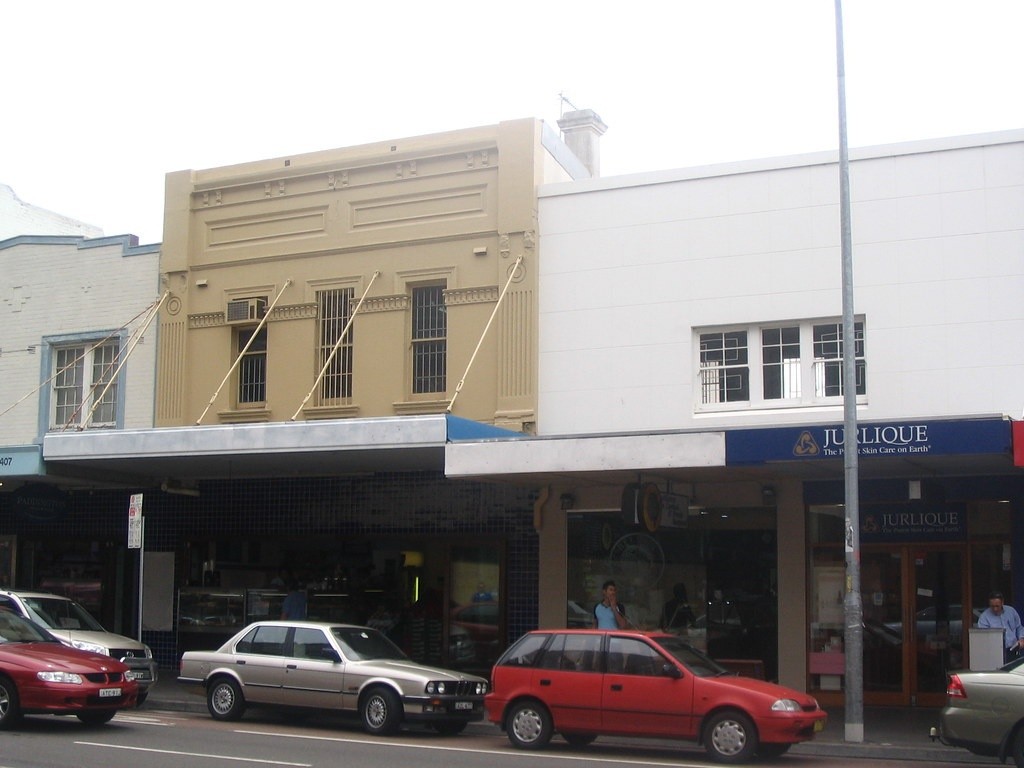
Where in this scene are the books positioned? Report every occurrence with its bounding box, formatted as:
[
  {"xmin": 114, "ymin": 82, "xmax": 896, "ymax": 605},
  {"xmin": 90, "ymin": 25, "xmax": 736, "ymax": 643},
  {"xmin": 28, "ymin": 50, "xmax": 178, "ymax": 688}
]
[{"xmin": 1010, "ymin": 642, "xmax": 1019, "ymax": 651}]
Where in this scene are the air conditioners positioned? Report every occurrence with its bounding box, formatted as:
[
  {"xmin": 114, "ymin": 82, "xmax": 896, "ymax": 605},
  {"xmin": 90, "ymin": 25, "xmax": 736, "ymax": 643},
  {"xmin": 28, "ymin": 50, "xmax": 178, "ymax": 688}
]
[{"xmin": 226, "ymin": 298, "xmax": 266, "ymax": 328}]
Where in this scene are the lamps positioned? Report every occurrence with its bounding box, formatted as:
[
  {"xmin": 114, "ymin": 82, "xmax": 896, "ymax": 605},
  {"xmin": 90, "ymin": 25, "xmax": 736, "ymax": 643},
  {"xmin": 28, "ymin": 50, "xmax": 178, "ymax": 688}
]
[
  {"xmin": 195, "ymin": 279, "xmax": 208, "ymax": 287},
  {"xmin": 473, "ymin": 246, "xmax": 488, "ymax": 256}
]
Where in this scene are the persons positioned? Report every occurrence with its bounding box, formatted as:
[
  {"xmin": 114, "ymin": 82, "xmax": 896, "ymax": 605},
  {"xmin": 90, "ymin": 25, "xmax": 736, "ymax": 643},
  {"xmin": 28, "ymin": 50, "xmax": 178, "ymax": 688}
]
[
  {"xmin": 186, "ymin": 567, "xmax": 300, "ymax": 625},
  {"xmin": 280, "ymin": 576, "xmax": 308, "ymax": 620},
  {"xmin": 977, "ymin": 590, "xmax": 1024, "ymax": 663},
  {"xmin": 707, "ymin": 586, "xmax": 742, "ymax": 658},
  {"xmin": 330, "ymin": 564, "xmax": 348, "ymax": 617},
  {"xmin": 592, "ymin": 580, "xmax": 627, "ymax": 629},
  {"xmin": 473, "ymin": 582, "xmax": 494, "ymax": 602}
]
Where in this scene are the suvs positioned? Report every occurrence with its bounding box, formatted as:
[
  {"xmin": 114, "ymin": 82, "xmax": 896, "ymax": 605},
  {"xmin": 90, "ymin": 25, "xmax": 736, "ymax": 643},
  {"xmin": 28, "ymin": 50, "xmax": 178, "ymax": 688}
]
[{"xmin": 0, "ymin": 588, "xmax": 160, "ymax": 709}]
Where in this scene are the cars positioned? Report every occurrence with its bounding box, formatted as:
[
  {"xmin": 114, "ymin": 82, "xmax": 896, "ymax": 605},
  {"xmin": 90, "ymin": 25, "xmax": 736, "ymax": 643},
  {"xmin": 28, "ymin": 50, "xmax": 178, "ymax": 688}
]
[
  {"xmin": 861, "ymin": 614, "xmax": 945, "ymax": 691},
  {"xmin": 177, "ymin": 619, "xmax": 488, "ymax": 738},
  {"xmin": 885, "ymin": 602, "xmax": 984, "ymax": 654},
  {"xmin": 481, "ymin": 627, "xmax": 829, "ymax": 765},
  {"xmin": 936, "ymin": 655, "xmax": 1024, "ymax": 768},
  {"xmin": 0, "ymin": 604, "xmax": 140, "ymax": 729}
]
